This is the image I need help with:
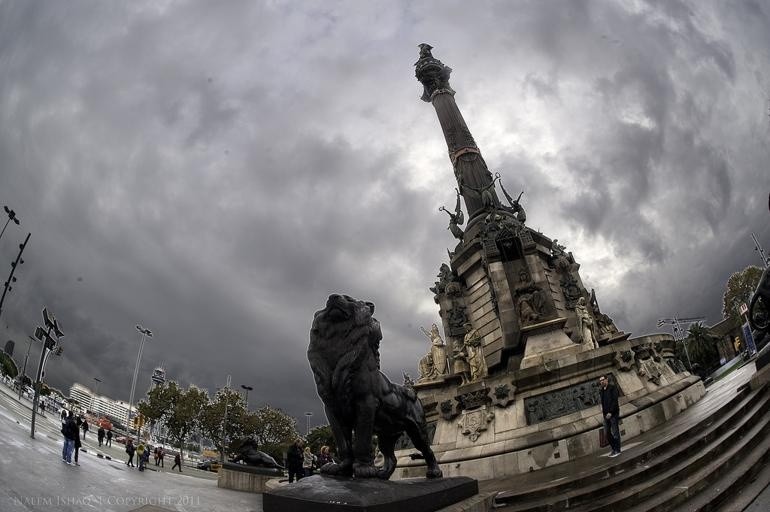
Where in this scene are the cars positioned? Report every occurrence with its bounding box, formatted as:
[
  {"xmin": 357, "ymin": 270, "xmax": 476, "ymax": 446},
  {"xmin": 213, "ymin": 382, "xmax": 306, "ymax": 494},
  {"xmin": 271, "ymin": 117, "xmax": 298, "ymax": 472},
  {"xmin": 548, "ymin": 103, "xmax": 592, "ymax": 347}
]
[
  {"xmin": 115, "ymin": 436, "xmax": 134, "ymax": 444},
  {"xmin": 196, "ymin": 458, "xmax": 221, "ymax": 471}
]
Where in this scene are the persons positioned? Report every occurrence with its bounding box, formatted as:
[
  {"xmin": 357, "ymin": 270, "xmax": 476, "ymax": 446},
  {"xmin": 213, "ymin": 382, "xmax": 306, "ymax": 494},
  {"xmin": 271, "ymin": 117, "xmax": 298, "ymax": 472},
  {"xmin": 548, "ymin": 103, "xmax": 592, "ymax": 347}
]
[
  {"xmin": 513, "ymin": 191, "xmax": 526, "ymax": 223},
  {"xmin": 40, "ymin": 401, "xmax": 45, "ymax": 415},
  {"xmin": 599, "ymin": 375, "xmax": 622, "ymax": 458},
  {"xmin": 452, "ymin": 339, "xmax": 470, "ymax": 388},
  {"xmin": 575, "ymin": 296, "xmax": 599, "ymax": 349},
  {"xmin": 443, "ymin": 206, "xmax": 464, "ymax": 241},
  {"xmin": 464, "ymin": 177, "xmax": 498, "ymax": 209},
  {"xmin": 61, "ymin": 410, "xmax": 183, "ymax": 472},
  {"xmin": 285, "ymin": 438, "xmax": 335, "ymax": 483},
  {"xmin": 463, "ymin": 322, "xmax": 487, "ymax": 384},
  {"xmin": 420, "ymin": 324, "xmax": 446, "ymax": 375},
  {"xmin": 446, "ymin": 300, "xmax": 469, "ymax": 335},
  {"xmin": 513, "ymin": 270, "xmax": 545, "ymax": 321}
]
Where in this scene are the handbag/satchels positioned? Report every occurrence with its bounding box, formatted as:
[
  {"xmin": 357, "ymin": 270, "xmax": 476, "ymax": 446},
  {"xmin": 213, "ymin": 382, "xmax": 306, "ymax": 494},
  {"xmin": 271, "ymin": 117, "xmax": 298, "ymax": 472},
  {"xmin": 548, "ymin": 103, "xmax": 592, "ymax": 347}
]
[{"xmin": 599, "ymin": 429, "xmax": 610, "ymax": 448}]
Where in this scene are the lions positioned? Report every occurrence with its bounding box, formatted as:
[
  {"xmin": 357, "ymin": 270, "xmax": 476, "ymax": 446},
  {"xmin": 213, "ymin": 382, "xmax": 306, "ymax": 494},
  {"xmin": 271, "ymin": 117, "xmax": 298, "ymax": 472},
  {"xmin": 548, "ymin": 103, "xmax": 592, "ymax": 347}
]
[
  {"xmin": 308, "ymin": 293, "xmax": 444, "ymax": 480},
  {"xmin": 228, "ymin": 437, "xmax": 285, "ymax": 472}
]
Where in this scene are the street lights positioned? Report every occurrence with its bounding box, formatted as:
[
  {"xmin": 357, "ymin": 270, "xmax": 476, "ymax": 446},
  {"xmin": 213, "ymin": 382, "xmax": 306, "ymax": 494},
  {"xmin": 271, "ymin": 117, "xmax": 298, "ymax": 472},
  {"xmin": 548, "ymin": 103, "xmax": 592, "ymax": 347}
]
[
  {"xmin": 0, "ymin": 205, "xmax": 22, "ymax": 238},
  {"xmin": 240, "ymin": 383, "xmax": 255, "ymax": 402},
  {"xmin": 0, "ymin": 233, "xmax": 32, "ymax": 310},
  {"xmin": 150, "ymin": 367, "xmax": 165, "ymax": 443},
  {"xmin": 29, "ymin": 307, "xmax": 65, "ymax": 438},
  {"xmin": 18, "ymin": 335, "xmax": 36, "ymax": 400},
  {"xmin": 127, "ymin": 324, "xmax": 155, "ymax": 410},
  {"xmin": 88, "ymin": 376, "xmax": 104, "ymax": 428},
  {"xmin": 751, "ymin": 233, "xmax": 770, "ymax": 267},
  {"xmin": 305, "ymin": 410, "xmax": 313, "ymax": 434},
  {"xmin": 672, "ymin": 318, "xmax": 695, "ymax": 376}
]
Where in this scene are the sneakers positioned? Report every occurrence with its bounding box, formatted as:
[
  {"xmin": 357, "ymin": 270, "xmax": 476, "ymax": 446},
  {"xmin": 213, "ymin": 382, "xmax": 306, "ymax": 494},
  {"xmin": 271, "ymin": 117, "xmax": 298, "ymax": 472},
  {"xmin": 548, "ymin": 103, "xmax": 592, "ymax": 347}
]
[{"xmin": 609, "ymin": 450, "xmax": 620, "ymax": 457}]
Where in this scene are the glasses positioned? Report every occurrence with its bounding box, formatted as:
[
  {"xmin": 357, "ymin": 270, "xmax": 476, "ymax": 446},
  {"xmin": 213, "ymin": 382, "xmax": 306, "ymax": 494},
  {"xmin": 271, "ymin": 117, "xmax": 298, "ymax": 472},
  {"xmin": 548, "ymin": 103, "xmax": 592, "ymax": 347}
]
[{"xmin": 599, "ymin": 379, "xmax": 605, "ymax": 382}]
